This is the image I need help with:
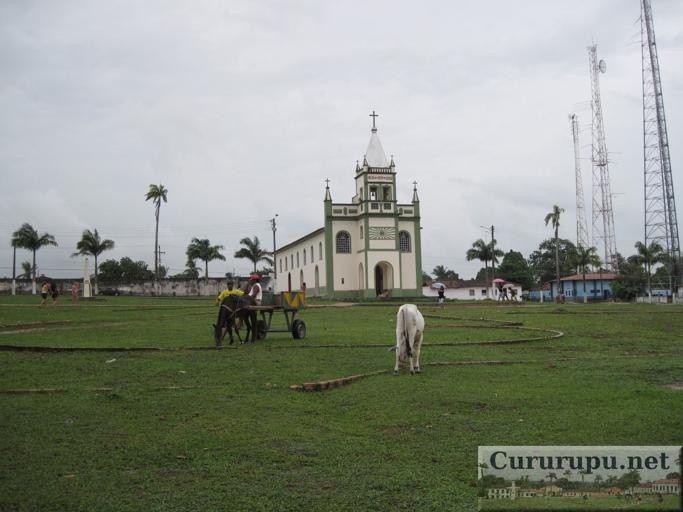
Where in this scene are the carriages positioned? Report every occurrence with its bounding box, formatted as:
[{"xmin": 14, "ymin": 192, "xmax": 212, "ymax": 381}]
[{"xmin": 211, "ymin": 290, "xmax": 305, "ymax": 348}]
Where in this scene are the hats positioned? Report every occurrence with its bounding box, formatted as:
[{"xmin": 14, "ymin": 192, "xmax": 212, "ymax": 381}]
[{"xmin": 249, "ymin": 274, "xmax": 258, "ymax": 280}]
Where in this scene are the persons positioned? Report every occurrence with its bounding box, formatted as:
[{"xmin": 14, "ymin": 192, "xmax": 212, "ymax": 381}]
[
  {"xmin": 300, "ymin": 282, "xmax": 306, "ymax": 293},
  {"xmin": 508, "ymin": 287, "xmax": 518, "ymax": 301},
  {"xmin": 437, "ymin": 286, "xmax": 445, "ymax": 310},
  {"xmin": 235, "ymin": 274, "xmax": 262, "ymax": 329},
  {"xmin": 496, "ymin": 283, "xmax": 504, "ymax": 301},
  {"xmin": 70, "ymin": 280, "xmax": 80, "ymax": 305},
  {"xmin": 38, "ymin": 278, "xmax": 67, "ymax": 308},
  {"xmin": 503, "ymin": 288, "xmax": 509, "ymax": 301},
  {"xmin": 215, "ymin": 280, "xmax": 244, "ymax": 328}
]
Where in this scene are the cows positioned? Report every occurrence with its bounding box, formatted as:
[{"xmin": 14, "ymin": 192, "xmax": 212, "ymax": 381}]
[{"xmin": 388, "ymin": 303, "xmax": 425, "ymax": 376}]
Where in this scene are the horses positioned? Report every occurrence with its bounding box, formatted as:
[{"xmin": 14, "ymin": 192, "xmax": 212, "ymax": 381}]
[{"xmin": 213, "ymin": 292, "xmax": 258, "ymax": 347}]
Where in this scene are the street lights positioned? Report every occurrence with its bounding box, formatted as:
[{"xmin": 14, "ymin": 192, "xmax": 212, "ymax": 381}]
[{"xmin": 479, "ymin": 224, "xmax": 496, "ymax": 301}]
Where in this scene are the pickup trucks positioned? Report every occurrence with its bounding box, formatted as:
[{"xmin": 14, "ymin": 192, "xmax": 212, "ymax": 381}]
[{"xmin": 99, "ymin": 287, "xmax": 123, "ymax": 296}]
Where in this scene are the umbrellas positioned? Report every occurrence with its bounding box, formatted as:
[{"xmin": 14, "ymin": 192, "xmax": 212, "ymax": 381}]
[
  {"xmin": 491, "ymin": 279, "xmax": 505, "ymax": 284},
  {"xmin": 431, "ymin": 282, "xmax": 445, "ymax": 289},
  {"xmin": 501, "ymin": 284, "xmax": 513, "ymax": 289}
]
